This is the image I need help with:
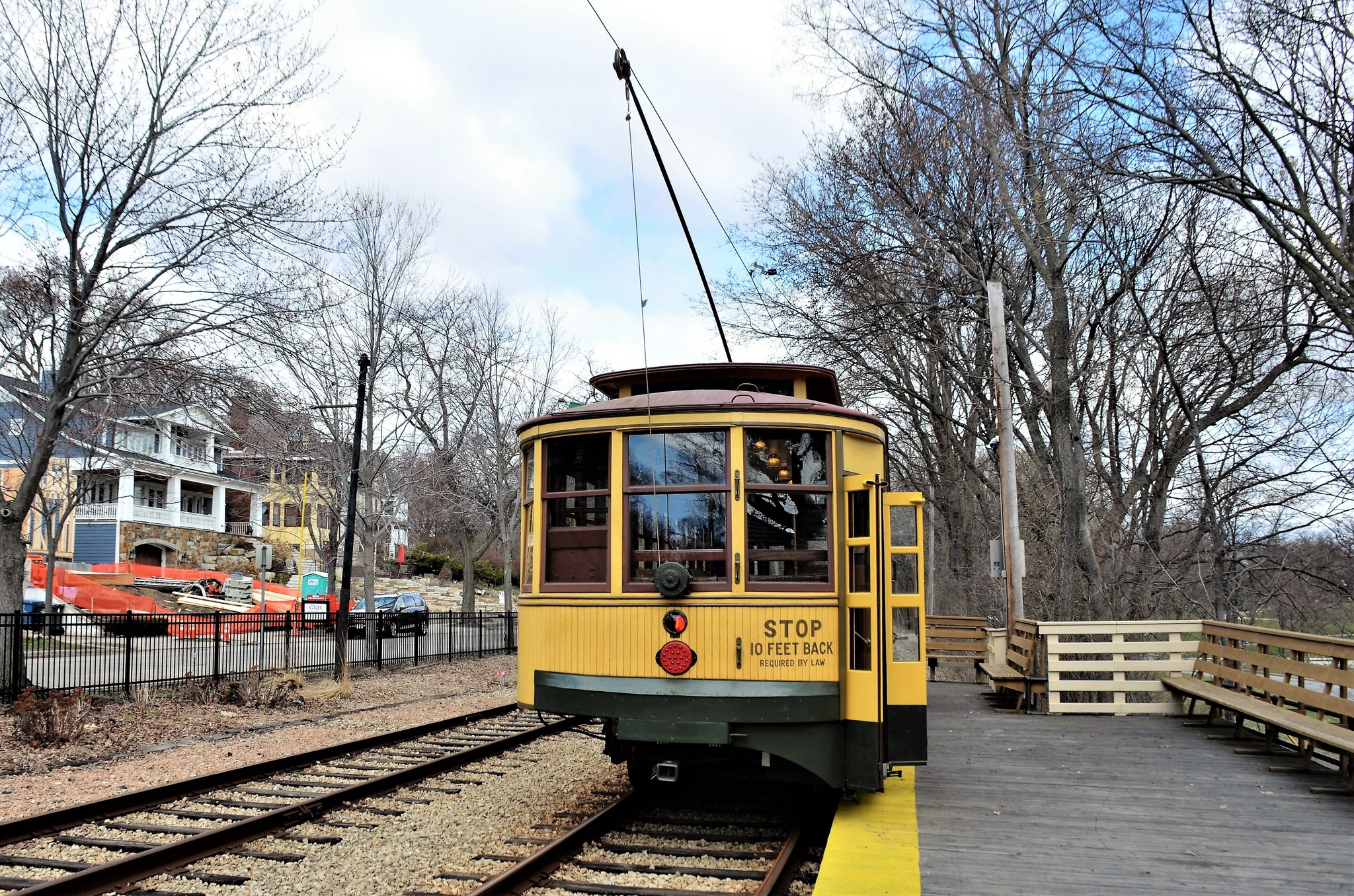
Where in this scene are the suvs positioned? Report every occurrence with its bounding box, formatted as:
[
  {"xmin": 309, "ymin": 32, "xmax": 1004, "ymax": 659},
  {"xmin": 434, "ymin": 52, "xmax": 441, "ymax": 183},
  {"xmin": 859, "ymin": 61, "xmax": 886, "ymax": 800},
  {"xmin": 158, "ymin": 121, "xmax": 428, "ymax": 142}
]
[{"xmin": 348, "ymin": 593, "xmax": 429, "ymax": 638}]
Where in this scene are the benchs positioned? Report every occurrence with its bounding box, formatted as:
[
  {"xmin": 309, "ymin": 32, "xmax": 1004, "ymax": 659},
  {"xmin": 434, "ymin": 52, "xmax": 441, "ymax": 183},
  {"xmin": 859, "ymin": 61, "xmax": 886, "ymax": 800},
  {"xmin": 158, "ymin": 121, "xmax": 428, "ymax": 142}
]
[
  {"xmin": 1160, "ymin": 621, "xmax": 1354, "ymax": 795},
  {"xmin": 979, "ymin": 619, "xmax": 1037, "ymax": 714},
  {"xmin": 925, "ymin": 614, "xmax": 985, "ymax": 680}
]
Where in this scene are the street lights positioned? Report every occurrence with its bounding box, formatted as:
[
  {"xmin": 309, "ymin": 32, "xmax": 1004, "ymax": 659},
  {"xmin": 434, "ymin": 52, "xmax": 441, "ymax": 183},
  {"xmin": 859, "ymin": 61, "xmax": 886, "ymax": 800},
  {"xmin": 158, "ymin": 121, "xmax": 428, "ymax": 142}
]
[{"xmin": 310, "ymin": 353, "xmax": 372, "ymax": 685}]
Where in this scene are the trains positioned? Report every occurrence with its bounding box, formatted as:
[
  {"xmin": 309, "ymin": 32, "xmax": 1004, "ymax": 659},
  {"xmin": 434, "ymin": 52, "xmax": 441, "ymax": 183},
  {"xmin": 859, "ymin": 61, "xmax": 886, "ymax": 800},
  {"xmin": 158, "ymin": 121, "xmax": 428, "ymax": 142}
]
[{"xmin": 515, "ymin": 362, "xmax": 930, "ymax": 808}]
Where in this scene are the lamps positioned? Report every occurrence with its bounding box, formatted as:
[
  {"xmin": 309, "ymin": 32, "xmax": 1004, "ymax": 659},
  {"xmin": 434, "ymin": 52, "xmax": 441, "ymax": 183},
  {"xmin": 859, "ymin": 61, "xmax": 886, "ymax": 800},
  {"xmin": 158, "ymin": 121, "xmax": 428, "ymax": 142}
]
[
  {"xmin": 766, "ymin": 454, "xmax": 781, "ymax": 469},
  {"xmin": 779, "ymin": 467, "xmax": 791, "ymax": 480},
  {"xmin": 754, "ymin": 436, "xmax": 766, "ymax": 449}
]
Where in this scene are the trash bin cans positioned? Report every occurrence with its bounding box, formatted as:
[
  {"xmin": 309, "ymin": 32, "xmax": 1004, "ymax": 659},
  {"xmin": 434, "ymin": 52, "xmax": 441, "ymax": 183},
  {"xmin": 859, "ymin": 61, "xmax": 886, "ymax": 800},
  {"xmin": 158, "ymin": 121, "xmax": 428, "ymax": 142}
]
[
  {"xmin": 31, "ymin": 602, "xmax": 65, "ymax": 631},
  {"xmin": 22, "ymin": 599, "xmax": 45, "ymax": 624}
]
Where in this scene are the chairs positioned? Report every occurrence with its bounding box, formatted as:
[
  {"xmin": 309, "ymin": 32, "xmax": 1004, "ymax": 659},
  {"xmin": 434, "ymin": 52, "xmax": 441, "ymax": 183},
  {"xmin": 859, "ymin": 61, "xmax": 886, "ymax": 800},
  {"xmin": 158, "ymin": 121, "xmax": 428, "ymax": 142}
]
[{"xmin": 397, "ymin": 565, "xmax": 408, "ymax": 579}]
